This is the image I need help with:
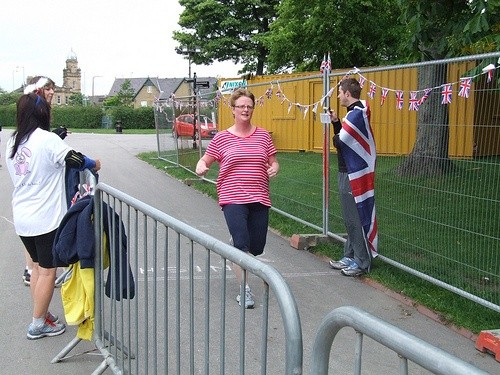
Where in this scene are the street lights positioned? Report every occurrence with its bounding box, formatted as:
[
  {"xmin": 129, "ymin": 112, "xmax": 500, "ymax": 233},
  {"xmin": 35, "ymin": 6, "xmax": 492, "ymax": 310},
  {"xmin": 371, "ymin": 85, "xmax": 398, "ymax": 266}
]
[
  {"xmin": 93, "ymin": 76, "xmax": 103, "ymax": 96},
  {"xmin": 13, "ymin": 65, "xmax": 25, "ymax": 91}
]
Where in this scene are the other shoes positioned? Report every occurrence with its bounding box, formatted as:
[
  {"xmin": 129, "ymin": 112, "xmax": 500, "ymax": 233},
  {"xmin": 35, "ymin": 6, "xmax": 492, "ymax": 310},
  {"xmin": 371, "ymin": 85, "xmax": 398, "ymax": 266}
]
[{"xmin": 22, "ymin": 268, "xmax": 58, "ymax": 288}]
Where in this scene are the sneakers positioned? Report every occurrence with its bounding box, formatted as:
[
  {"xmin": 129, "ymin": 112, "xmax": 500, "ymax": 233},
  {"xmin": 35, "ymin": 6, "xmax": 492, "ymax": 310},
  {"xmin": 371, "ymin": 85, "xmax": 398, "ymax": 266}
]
[
  {"xmin": 341, "ymin": 263, "xmax": 367, "ymax": 277},
  {"xmin": 235, "ymin": 287, "xmax": 255, "ymax": 309},
  {"xmin": 46, "ymin": 312, "xmax": 60, "ymax": 325},
  {"xmin": 26, "ymin": 319, "xmax": 65, "ymax": 340},
  {"xmin": 329, "ymin": 257, "xmax": 355, "ymax": 270}
]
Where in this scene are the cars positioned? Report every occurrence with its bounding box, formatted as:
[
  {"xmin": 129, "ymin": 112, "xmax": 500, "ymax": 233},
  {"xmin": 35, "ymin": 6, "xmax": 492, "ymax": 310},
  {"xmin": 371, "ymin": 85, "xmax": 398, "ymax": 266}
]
[{"xmin": 172, "ymin": 114, "xmax": 218, "ymax": 139}]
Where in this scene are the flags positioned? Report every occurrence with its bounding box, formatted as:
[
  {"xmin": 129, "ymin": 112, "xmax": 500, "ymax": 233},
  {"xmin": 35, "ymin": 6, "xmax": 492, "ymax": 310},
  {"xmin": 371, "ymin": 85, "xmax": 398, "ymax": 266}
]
[
  {"xmin": 338, "ymin": 99, "xmax": 379, "ymax": 259},
  {"xmin": 359, "ymin": 71, "xmax": 492, "ymax": 111},
  {"xmin": 154, "ymin": 60, "xmax": 331, "ymax": 119}
]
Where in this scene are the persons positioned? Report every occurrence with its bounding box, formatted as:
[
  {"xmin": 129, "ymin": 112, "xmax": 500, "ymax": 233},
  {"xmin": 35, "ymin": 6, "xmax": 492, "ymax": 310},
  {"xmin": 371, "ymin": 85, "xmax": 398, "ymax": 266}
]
[
  {"xmin": 196, "ymin": 90, "xmax": 280, "ymax": 309},
  {"xmin": 23, "ymin": 76, "xmax": 63, "ymax": 288},
  {"xmin": 327, "ymin": 78, "xmax": 371, "ymax": 276},
  {"xmin": 5, "ymin": 94, "xmax": 101, "ymax": 339}
]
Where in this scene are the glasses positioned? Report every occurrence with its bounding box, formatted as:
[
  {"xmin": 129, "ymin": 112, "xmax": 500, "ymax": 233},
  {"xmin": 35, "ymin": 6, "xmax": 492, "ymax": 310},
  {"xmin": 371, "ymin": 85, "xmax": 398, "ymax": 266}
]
[{"xmin": 232, "ymin": 104, "xmax": 254, "ymax": 110}]
